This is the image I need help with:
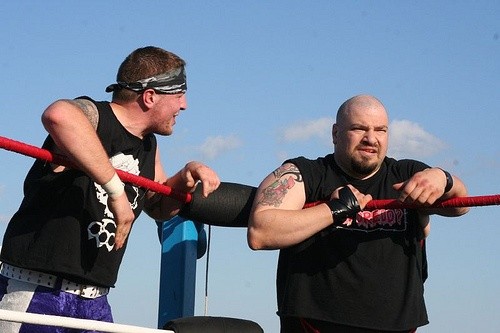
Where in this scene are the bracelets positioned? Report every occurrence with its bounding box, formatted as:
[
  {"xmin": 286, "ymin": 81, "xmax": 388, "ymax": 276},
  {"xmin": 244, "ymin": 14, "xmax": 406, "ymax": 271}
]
[
  {"xmin": 437, "ymin": 167, "xmax": 453, "ymax": 194},
  {"xmin": 102, "ymin": 171, "xmax": 125, "ymax": 197}
]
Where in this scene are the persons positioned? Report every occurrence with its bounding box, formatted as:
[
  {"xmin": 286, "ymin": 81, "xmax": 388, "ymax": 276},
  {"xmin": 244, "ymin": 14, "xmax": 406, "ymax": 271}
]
[
  {"xmin": 0, "ymin": 46, "xmax": 220, "ymax": 333},
  {"xmin": 248, "ymin": 94, "xmax": 470, "ymax": 333}
]
